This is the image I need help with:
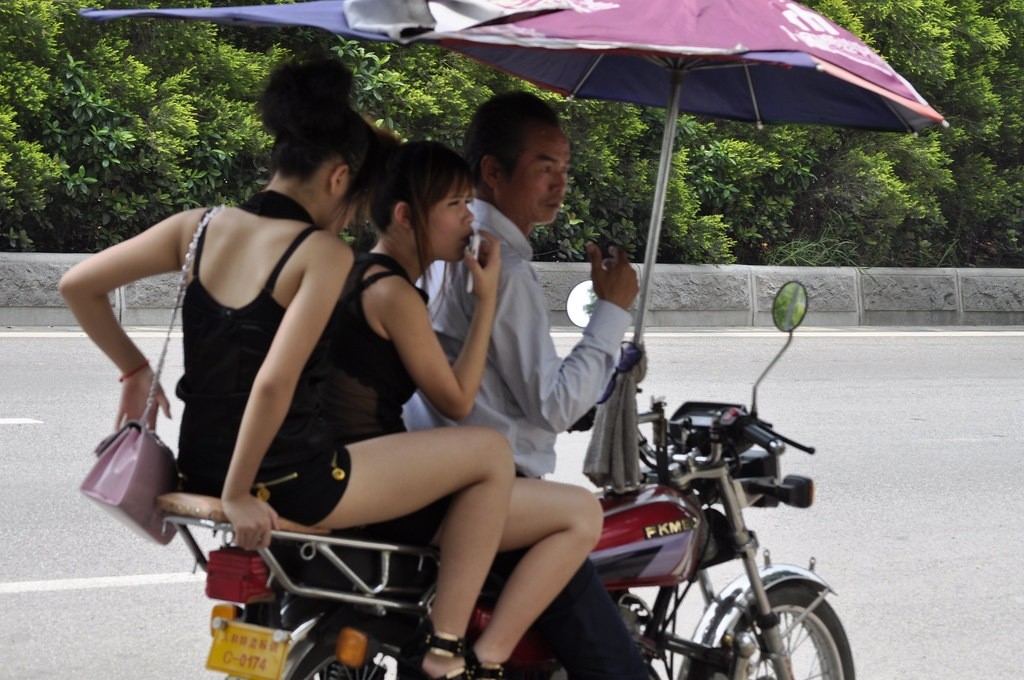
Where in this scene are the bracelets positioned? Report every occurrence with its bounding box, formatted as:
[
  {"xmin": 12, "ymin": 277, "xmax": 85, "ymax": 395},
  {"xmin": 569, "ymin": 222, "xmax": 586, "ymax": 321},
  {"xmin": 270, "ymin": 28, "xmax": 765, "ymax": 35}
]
[{"xmin": 119, "ymin": 359, "xmax": 150, "ymax": 382}]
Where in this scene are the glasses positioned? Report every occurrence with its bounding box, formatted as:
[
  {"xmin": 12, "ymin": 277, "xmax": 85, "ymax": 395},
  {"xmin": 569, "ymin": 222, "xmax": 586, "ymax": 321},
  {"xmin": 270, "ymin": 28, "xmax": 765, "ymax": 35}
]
[{"xmin": 595, "ymin": 341, "xmax": 643, "ymax": 404}]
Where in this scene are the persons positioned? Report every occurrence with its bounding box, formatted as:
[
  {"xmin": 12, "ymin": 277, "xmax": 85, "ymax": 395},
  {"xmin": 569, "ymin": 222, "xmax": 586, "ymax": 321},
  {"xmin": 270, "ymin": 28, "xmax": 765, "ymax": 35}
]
[
  {"xmin": 399, "ymin": 89, "xmax": 670, "ymax": 680},
  {"xmin": 56, "ymin": 54, "xmax": 515, "ymax": 680},
  {"xmin": 331, "ymin": 127, "xmax": 604, "ymax": 680}
]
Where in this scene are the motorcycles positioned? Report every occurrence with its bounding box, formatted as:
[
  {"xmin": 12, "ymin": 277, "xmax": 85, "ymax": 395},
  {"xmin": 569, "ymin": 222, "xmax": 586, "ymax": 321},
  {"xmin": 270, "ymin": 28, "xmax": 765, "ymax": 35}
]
[{"xmin": 161, "ymin": 279, "xmax": 856, "ymax": 680}]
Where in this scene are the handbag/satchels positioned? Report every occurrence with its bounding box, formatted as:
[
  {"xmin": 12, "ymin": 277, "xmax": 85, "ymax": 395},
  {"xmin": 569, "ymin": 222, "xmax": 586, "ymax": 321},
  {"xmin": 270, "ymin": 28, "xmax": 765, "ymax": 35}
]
[{"xmin": 81, "ymin": 420, "xmax": 180, "ymax": 547}]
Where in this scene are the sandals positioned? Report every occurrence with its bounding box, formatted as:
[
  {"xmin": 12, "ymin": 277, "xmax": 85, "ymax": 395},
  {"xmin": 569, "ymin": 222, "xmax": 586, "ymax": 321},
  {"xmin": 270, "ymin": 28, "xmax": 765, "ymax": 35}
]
[
  {"xmin": 466, "ymin": 648, "xmax": 506, "ymax": 680},
  {"xmin": 418, "ymin": 634, "xmax": 468, "ymax": 680}
]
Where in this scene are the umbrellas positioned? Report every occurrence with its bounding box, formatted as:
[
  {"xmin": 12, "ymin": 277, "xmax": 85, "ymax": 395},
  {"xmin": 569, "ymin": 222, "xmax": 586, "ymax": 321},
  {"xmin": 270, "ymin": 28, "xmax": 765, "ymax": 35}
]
[{"xmin": 81, "ymin": 0, "xmax": 950, "ymax": 345}]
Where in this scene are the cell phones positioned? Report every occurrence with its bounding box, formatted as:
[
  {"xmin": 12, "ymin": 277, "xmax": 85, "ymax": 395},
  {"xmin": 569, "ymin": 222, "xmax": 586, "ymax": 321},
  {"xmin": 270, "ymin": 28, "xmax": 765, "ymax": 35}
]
[{"xmin": 465, "ymin": 235, "xmax": 481, "ymax": 294}]
